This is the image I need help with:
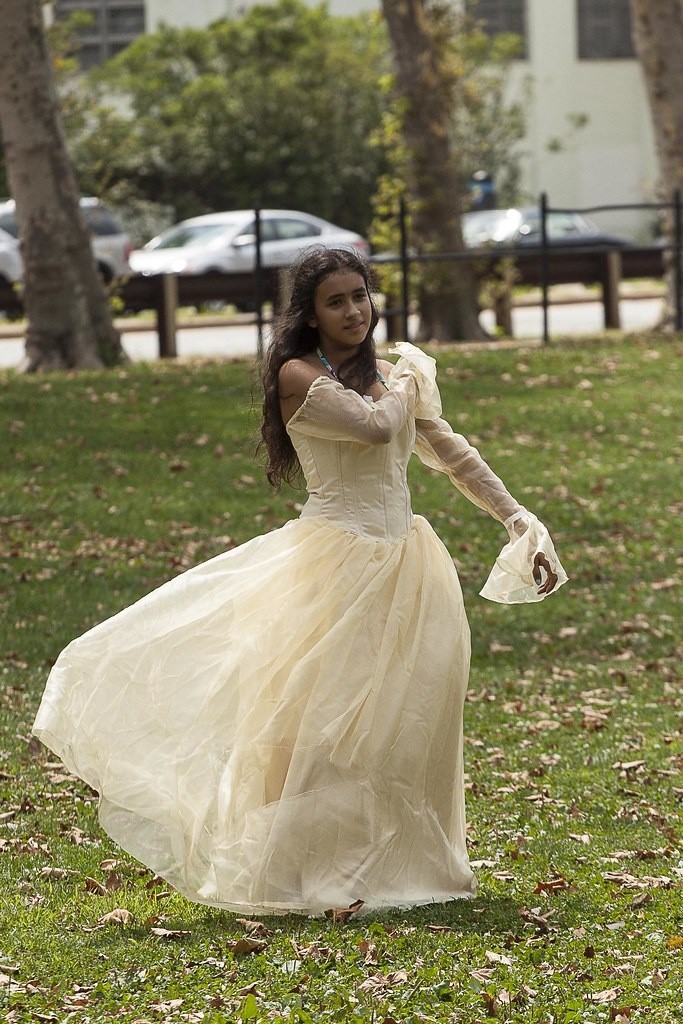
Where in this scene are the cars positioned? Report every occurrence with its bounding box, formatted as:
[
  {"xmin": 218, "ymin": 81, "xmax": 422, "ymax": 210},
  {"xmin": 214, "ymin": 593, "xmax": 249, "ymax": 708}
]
[
  {"xmin": 459, "ymin": 207, "xmax": 635, "ymax": 246},
  {"xmin": 127, "ymin": 207, "xmax": 370, "ymax": 275}
]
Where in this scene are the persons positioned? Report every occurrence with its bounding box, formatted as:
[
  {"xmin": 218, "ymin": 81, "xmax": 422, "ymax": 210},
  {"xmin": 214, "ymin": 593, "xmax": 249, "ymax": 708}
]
[
  {"xmin": 249, "ymin": 244, "xmax": 559, "ymax": 919},
  {"xmin": 469, "ymin": 170, "xmax": 498, "ymax": 211}
]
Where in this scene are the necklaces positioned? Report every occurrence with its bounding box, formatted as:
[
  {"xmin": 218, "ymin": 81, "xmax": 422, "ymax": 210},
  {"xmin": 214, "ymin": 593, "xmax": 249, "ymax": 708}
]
[{"xmin": 314, "ymin": 345, "xmax": 390, "ymax": 402}]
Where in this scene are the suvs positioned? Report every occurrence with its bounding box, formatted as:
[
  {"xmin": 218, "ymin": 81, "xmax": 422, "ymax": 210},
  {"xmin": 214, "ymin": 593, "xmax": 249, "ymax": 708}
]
[{"xmin": 0, "ymin": 197, "xmax": 132, "ymax": 288}]
